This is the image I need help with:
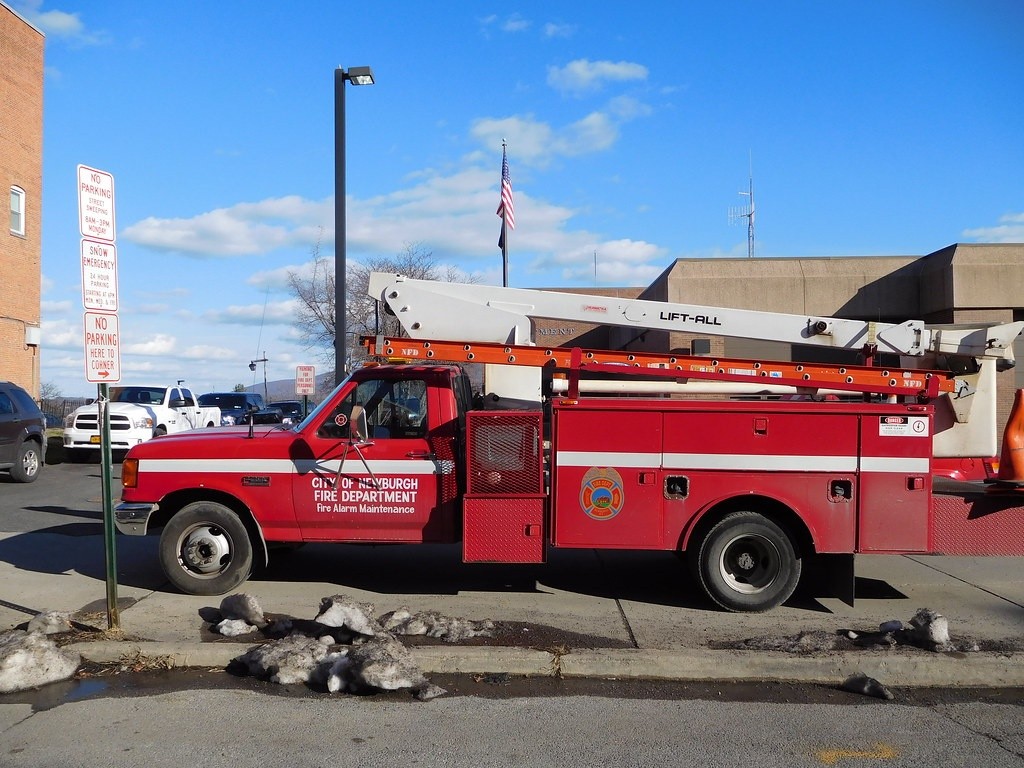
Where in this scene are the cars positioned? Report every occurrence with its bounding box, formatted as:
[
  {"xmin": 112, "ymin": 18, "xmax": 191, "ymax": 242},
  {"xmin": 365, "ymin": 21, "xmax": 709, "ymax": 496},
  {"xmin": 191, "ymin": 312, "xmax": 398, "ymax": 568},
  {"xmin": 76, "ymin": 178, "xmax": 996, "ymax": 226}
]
[
  {"xmin": 0, "ymin": 381, "xmax": 49, "ymax": 482},
  {"xmin": 268, "ymin": 400, "xmax": 318, "ymax": 425}
]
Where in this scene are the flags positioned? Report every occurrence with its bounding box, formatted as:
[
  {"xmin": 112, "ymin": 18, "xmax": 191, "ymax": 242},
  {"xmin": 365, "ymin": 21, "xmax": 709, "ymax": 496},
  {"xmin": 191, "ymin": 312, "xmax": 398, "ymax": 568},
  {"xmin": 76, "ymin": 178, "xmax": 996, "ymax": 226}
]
[{"xmin": 495, "ymin": 147, "xmax": 515, "ymax": 230}]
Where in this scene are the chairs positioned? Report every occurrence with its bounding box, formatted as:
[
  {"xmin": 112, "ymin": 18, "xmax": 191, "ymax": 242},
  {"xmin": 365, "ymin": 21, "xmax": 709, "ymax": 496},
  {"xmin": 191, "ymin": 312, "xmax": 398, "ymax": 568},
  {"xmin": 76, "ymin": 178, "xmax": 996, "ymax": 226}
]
[
  {"xmin": 128, "ymin": 391, "xmax": 138, "ymax": 401},
  {"xmin": 139, "ymin": 392, "xmax": 151, "ymax": 402}
]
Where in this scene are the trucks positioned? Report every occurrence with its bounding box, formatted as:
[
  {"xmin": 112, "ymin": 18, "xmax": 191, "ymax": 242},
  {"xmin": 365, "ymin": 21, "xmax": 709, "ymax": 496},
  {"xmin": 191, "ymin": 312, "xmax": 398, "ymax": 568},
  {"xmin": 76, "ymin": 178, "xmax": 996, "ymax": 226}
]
[{"xmin": 115, "ymin": 280, "xmax": 1024, "ymax": 613}]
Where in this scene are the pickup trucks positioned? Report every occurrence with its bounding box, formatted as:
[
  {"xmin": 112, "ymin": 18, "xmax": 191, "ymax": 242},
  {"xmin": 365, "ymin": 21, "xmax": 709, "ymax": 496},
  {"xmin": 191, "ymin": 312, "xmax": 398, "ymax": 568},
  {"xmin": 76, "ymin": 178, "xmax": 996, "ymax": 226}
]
[
  {"xmin": 61, "ymin": 380, "xmax": 223, "ymax": 463},
  {"xmin": 196, "ymin": 391, "xmax": 283, "ymax": 426}
]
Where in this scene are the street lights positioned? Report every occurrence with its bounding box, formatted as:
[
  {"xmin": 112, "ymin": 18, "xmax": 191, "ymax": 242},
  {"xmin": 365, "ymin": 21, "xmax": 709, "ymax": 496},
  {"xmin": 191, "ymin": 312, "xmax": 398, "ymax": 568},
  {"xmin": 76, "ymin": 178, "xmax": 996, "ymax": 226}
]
[
  {"xmin": 248, "ymin": 352, "xmax": 269, "ymax": 412},
  {"xmin": 332, "ymin": 66, "xmax": 377, "ymax": 411}
]
